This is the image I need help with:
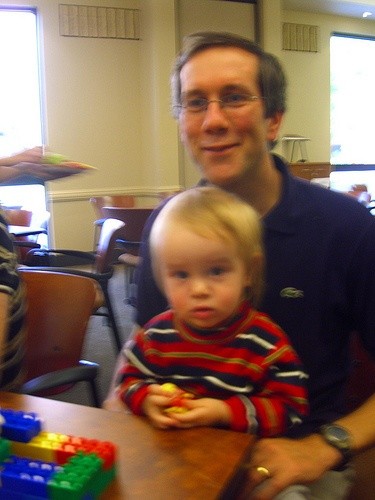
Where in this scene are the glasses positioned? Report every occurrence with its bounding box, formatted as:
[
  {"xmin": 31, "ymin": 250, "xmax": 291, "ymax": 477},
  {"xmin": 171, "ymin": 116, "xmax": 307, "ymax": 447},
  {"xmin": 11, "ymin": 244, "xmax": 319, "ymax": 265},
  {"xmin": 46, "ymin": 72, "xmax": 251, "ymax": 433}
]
[{"xmin": 175, "ymin": 92, "xmax": 265, "ymax": 113}]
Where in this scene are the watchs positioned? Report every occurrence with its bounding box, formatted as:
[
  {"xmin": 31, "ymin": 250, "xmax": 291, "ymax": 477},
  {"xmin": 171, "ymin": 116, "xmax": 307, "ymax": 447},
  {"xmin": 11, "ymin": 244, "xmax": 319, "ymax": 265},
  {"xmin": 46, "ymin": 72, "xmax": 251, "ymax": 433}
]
[{"xmin": 316, "ymin": 420, "xmax": 355, "ymax": 473}]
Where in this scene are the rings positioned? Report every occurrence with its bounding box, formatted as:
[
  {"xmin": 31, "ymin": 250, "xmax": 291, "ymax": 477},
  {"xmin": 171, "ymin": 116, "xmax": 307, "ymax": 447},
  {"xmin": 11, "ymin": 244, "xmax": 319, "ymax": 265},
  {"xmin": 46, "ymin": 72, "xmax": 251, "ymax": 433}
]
[{"xmin": 256, "ymin": 464, "xmax": 270, "ymax": 475}]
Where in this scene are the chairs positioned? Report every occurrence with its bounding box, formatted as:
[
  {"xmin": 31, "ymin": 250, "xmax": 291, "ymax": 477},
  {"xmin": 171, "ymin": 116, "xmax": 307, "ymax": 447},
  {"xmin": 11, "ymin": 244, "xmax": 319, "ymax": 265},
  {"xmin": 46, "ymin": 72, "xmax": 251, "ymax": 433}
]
[
  {"xmin": 9, "ymin": 265, "xmax": 100, "ymax": 409},
  {"xmin": 1, "ymin": 210, "xmax": 48, "ymax": 261},
  {"xmin": 87, "ymin": 194, "xmax": 136, "ymax": 272},
  {"xmin": 24, "ymin": 247, "xmax": 122, "ymax": 352},
  {"xmin": 101, "ymin": 205, "xmax": 158, "ymax": 308}
]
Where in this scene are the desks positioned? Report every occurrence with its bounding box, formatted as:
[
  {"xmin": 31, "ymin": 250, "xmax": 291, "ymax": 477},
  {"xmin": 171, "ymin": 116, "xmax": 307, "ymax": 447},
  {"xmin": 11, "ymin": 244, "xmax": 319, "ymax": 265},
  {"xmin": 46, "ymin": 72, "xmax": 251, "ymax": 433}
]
[{"xmin": 0, "ymin": 389, "xmax": 260, "ymax": 500}]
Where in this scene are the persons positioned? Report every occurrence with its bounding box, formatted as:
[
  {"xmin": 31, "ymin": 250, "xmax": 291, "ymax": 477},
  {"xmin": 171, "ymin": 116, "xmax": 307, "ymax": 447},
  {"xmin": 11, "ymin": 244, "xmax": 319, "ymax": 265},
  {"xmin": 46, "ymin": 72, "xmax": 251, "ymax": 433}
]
[
  {"xmin": 105, "ymin": 30, "xmax": 375, "ymax": 500},
  {"xmin": 0, "ymin": 211, "xmax": 30, "ymax": 394},
  {"xmin": 113, "ymin": 185, "xmax": 308, "ymax": 500}
]
